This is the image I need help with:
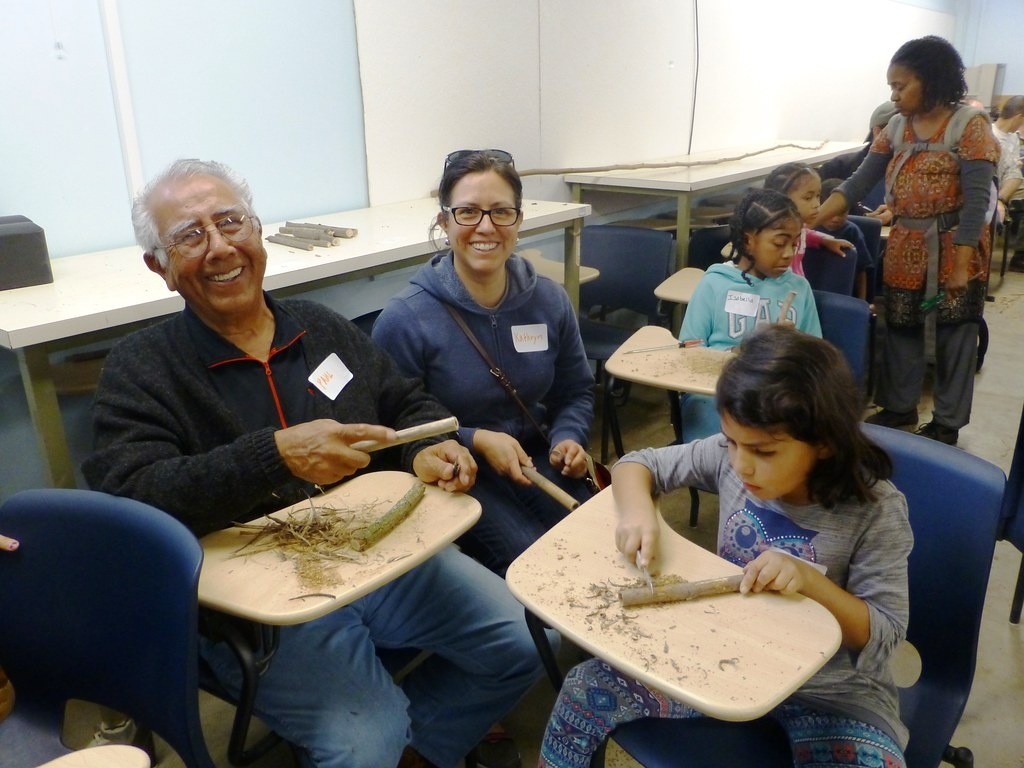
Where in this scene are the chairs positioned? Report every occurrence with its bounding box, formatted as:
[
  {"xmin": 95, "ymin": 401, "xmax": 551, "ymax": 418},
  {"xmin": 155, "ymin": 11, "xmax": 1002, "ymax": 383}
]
[
  {"xmin": 582, "ymin": 164, "xmax": 1024, "ymax": 768},
  {"xmin": 0, "ymin": 490, "xmax": 444, "ymax": 768}
]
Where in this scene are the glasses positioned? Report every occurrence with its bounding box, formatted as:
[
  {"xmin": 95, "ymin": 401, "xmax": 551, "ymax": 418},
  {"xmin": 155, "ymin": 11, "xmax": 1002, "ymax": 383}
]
[
  {"xmin": 152, "ymin": 213, "xmax": 255, "ymax": 259},
  {"xmin": 444, "ymin": 149, "xmax": 515, "ymax": 173},
  {"xmin": 442, "ymin": 206, "xmax": 521, "ymax": 226}
]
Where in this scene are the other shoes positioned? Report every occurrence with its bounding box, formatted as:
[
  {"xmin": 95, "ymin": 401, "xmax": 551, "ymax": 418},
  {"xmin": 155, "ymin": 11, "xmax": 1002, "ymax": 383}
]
[
  {"xmin": 476, "ymin": 723, "xmax": 522, "ymax": 768},
  {"xmin": 1008, "ymin": 254, "xmax": 1024, "ymax": 272},
  {"xmin": 914, "ymin": 420, "xmax": 958, "ymax": 447},
  {"xmin": 864, "ymin": 409, "xmax": 919, "ymax": 432}
]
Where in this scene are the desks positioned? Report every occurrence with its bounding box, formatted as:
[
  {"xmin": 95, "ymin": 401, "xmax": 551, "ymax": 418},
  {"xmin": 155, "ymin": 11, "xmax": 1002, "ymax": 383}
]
[
  {"xmin": 708, "ymin": 193, "xmax": 745, "ymax": 208},
  {"xmin": 1, "ymin": 192, "xmax": 591, "ymax": 489},
  {"xmin": 605, "ymin": 326, "xmax": 736, "ymax": 397},
  {"xmin": 519, "ymin": 248, "xmax": 601, "ymax": 288},
  {"xmin": 606, "ymin": 219, "xmax": 730, "ymax": 234},
  {"xmin": 51, "ymin": 357, "xmax": 107, "ymax": 397},
  {"xmin": 505, "ymin": 483, "xmax": 842, "ymax": 722},
  {"xmin": 661, "ymin": 205, "xmax": 735, "ymax": 219},
  {"xmin": 654, "ymin": 267, "xmax": 705, "ymax": 304},
  {"xmin": 195, "ymin": 470, "xmax": 483, "ymax": 676},
  {"xmin": 565, "ymin": 139, "xmax": 872, "ymax": 338}
]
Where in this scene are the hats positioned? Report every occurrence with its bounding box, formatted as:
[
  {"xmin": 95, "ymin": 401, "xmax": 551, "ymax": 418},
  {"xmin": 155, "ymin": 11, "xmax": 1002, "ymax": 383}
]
[{"xmin": 864, "ymin": 104, "xmax": 899, "ymax": 142}]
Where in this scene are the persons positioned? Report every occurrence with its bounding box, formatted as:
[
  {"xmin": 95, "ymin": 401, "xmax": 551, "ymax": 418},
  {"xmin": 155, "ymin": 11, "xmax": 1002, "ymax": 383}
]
[
  {"xmin": 82, "ymin": 156, "xmax": 564, "ymax": 768},
  {"xmin": 815, "ymin": 177, "xmax": 877, "ymax": 303},
  {"xmin": 990, "ymin": 94, "xmax": 1023, "ymax": 273},
  {"xmin": 765, "ymin": 161, "xmax": 856, "ymax": 278},
  {"xmin": 536, "ymin": 323, "xmax": 917, "ymax": 767},
  {"xmin": 812, "ymin": 97, "xmax": 902, "ymax": 228},
  {"xmin": 675, "ymin": 186, "xmax": 825, "ymax": 444},
  {"xmin": 368, "ymin": 150, "xmax": 598, "ymax": 583},
  {"xmin": 809, "ymin": 35, "xmax": 1002, "ymax": 445}
]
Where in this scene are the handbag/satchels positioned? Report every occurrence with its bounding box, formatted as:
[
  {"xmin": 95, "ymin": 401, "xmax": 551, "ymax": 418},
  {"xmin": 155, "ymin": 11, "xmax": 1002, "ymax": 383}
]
[{"xmin": 585, "ymin": 452, "xmax": 612, "ymax": 495}]
